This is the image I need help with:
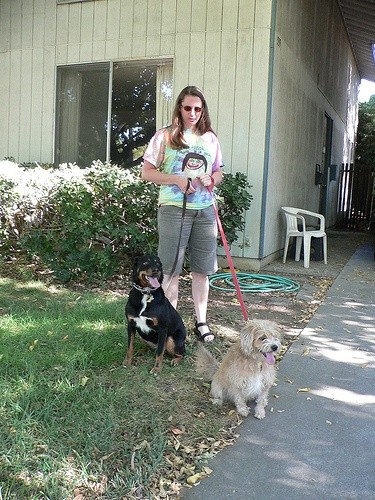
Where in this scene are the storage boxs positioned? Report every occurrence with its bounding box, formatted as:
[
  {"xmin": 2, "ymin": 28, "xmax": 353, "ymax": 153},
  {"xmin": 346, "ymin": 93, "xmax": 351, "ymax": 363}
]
[{"xmin": 315, "ymin": 164, "xmax": 323, "ymax": 185}]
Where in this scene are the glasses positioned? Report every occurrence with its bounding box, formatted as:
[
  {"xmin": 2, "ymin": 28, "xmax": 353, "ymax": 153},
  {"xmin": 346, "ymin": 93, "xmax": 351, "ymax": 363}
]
[{"xmin": 180, "ymin": 106, "xmax": 202, "ymax": 113}]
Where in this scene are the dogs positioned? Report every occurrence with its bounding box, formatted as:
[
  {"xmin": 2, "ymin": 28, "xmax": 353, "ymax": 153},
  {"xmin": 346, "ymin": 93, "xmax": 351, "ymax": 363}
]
[
  {"xmin": 191, "ymin": 318, "xmax": 283, "ymax": 420},
  {"xmin": 122, "ymin": 255, "xmax": 187, "ymax": 377}
]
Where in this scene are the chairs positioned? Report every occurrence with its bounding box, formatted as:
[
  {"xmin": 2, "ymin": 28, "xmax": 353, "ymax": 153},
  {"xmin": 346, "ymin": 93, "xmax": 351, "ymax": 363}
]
[{"xmin": 281, "ymin": 206, "xmax": 328, "ymax": 269}]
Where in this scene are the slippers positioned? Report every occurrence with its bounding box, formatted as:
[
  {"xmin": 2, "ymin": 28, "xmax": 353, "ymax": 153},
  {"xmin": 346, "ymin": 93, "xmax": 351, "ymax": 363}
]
[{"xmin": 194, "ymin": 320, "xmax": 216, "ymax": 344}]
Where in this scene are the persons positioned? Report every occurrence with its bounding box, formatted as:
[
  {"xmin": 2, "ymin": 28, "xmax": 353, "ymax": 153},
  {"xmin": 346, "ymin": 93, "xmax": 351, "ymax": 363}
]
[{"xmin": 141, "ymin": 85, "xmax": 225, "ymax": 343}]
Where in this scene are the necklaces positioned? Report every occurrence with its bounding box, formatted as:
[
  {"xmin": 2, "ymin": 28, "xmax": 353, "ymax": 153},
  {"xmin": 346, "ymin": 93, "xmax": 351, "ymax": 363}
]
[{"xmin": 182, "ymin": 127, "xmax": 197, "ymax": 134}]
